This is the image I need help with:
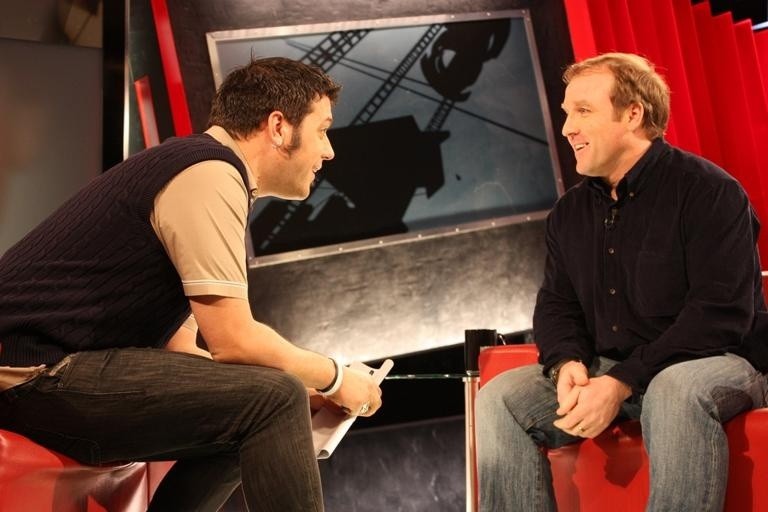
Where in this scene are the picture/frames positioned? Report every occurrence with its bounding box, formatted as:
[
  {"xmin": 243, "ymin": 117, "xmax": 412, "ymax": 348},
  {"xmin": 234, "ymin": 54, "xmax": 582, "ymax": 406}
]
[{"xmin": 198, "ymin": 10, "xmax": 565, "ymax": 271}]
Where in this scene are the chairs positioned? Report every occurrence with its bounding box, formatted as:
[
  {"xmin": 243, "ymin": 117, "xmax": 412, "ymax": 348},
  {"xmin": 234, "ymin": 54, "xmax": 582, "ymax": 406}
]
[{"xmin": 474, "ymin": 269, "xmax": 765, "ymax": 511}]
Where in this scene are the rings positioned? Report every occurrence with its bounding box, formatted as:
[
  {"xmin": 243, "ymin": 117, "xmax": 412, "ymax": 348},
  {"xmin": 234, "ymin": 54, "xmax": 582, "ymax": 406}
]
[
  {"xmin": 577, "ymin": 425, "xmax": 586, "ymax": 433},
  {"xmin": 361, "ymin": 401, "xmax": 371, "ymax": 413}
]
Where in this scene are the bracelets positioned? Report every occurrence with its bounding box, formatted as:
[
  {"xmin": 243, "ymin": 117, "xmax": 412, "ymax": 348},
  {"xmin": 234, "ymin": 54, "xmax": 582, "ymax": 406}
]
[
  {"xmin": 549, "ymin": 357, "xmax": 571, "ymax": 388},
  {"xmin": 316, "ymin": 357, "xmax": 343, "ymax": 396}
]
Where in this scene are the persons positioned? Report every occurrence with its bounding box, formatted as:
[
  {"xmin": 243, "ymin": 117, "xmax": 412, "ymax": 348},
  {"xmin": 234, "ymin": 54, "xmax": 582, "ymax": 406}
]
[
  {"xmin": 0, "ymin": 58, "xmax": 381, "ymax": 510},
  {"xmin": 475, "ymin": 52, "xmax": 767, "ymax": 510}
]
